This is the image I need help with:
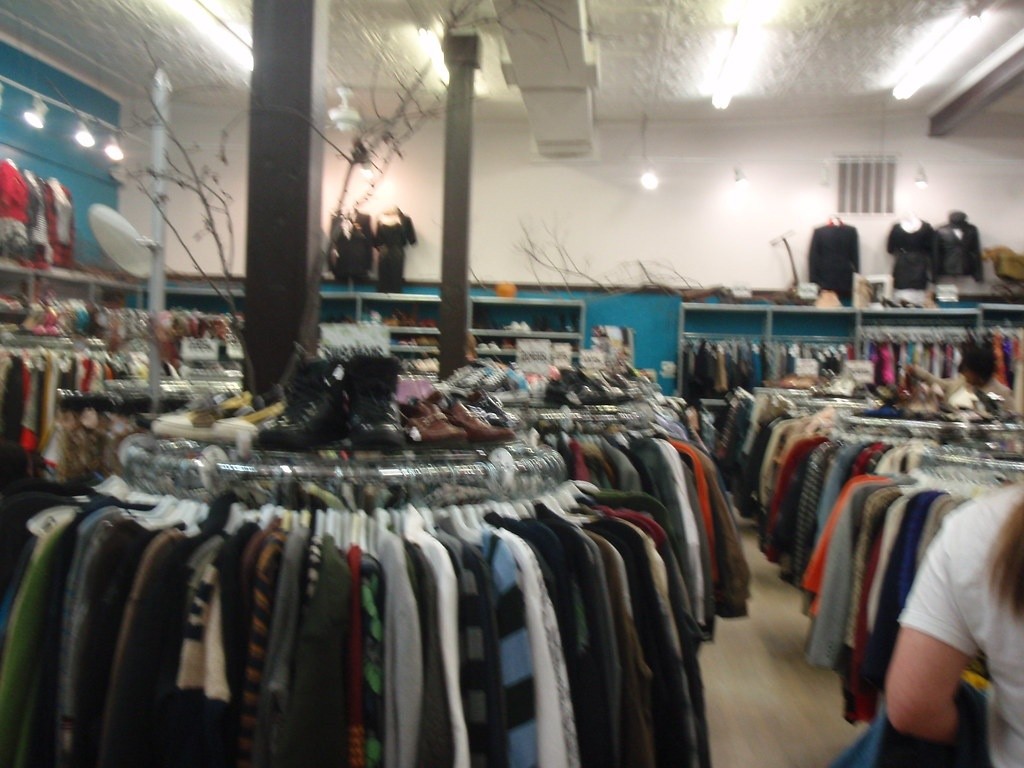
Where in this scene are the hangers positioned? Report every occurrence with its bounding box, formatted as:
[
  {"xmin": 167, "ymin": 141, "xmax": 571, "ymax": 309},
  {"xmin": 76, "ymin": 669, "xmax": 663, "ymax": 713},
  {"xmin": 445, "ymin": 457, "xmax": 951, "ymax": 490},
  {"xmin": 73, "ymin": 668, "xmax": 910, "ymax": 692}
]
[
  {"xmin": 0, "ymin": 332, "xmax": 689, "ymax": 553},
  {"xmin": 681, "ymin": 326, "xmax": 1024, "ymax": 502}
]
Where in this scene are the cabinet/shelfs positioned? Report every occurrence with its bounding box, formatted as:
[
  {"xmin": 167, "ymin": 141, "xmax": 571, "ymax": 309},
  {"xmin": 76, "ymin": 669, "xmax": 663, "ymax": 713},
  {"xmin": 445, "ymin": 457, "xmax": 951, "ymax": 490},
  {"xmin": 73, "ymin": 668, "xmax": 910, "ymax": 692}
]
[
  {"xmin": 0, "ymin": 257, "xmax": 586, "ymax": 370},
  {"xmin": 677, "ymin": 304, "xmax": 1024, "ymax": 406}
]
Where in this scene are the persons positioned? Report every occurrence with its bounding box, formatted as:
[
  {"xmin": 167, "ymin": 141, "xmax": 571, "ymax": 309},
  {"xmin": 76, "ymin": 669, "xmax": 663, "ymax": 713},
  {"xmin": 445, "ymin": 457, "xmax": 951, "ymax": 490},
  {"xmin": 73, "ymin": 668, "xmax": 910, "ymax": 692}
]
[
  {"xmin": 886, "ymin": 214, "xmax": 936, "ymax": 304},
  {"xmin": 374, "ymin": 207, "xmax": 415, "ymax": 292},
  {"xmin": 0, "ymin": 159, "xmax": 74, "ymax": 267},
  {"xmin": 808, "ymin": 218, "xmax": 858, "ymax": 299},
  {"xmin": 329, "ymin": 208, "xmax": 372, "ymax": 284},
  {"xmin": 938, "ymin": 212, "xmax": 981, "ymax": 283},
  {"xmin": 885, "ymin": 485, "xmax": 1024, "ymax": 768}
]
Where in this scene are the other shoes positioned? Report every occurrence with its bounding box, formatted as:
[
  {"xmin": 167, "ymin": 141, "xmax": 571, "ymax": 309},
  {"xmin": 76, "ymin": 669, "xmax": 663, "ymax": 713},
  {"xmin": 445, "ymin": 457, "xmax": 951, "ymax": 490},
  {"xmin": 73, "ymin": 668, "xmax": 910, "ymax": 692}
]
[
  {"xmin": 397, "ymin": 402, "xmax": 466, "ymax": 446},
  {"xmin": 215, "ymin": 401, "xmax": 288, "ymax": 431},
  {"xmin": 174, "ymin": 392, "xmax": 252, "ymax": 429},
  {"xmin": 453, "ymin": 390, "xmax": 521, "ymax": 429},
  {"xmin": 429, "ymin": 391, "xmax": 515, "ymax": 443}
]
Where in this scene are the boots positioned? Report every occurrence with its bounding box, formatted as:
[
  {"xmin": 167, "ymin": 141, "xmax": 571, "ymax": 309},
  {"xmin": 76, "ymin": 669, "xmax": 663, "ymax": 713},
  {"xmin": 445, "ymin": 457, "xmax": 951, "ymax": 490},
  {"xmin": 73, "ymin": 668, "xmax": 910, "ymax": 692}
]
[
  {"xmin": 341, "ymin": 352, "xmax": 407, "ymax": 448},
  {"xmin": 260, "ymin": 359, "xmax": 349, "ymax": 449}
]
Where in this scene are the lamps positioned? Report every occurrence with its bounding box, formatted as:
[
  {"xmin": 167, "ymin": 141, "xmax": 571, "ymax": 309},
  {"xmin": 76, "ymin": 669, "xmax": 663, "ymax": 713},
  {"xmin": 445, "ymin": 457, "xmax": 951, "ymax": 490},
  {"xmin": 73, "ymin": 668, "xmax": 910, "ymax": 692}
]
[
  {"xmin": 105, "ymin": 134, "xmax": 124, "ymax": 162},
  {"xmin": 22, "ymin": 96, "xmax": 49, "ymax": 129},
  {"xmin": 73, "ymin": 120, "xmax": 96, "ymax": 148},
  {"xmin": 329, "ymin": 86, "xmax": 363, "ymax": 134}
]
